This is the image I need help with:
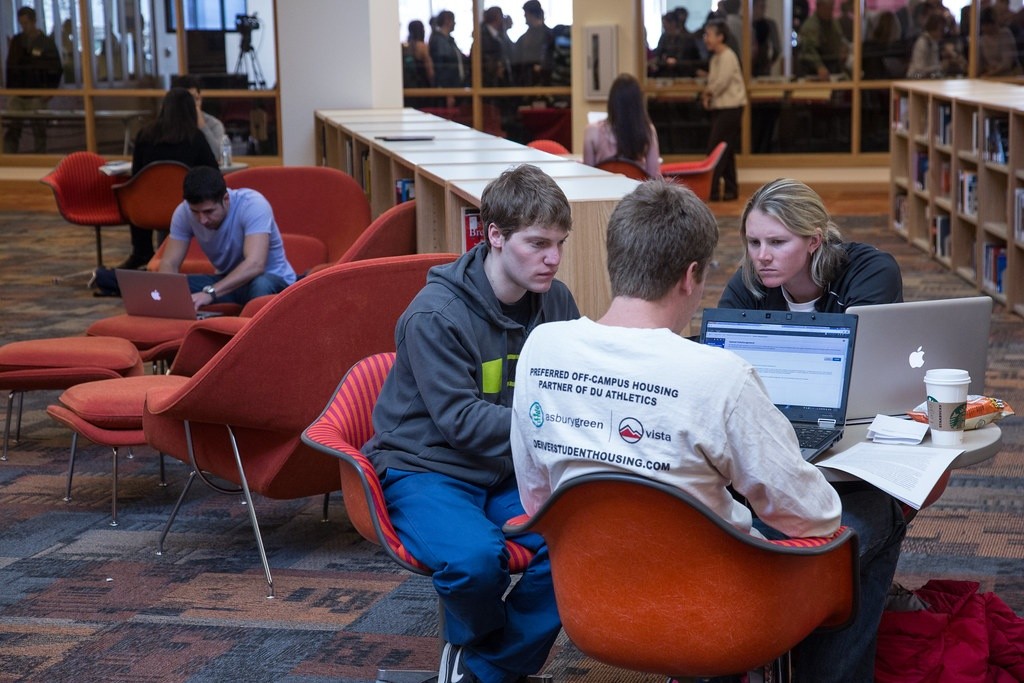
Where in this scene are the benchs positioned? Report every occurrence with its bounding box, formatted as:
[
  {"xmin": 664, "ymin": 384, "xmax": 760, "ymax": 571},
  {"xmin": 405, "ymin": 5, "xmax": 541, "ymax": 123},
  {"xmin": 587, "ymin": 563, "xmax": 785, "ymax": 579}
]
[{"xmin": 0, "ymin": 110, "xmax": 149, "ymax": 161}]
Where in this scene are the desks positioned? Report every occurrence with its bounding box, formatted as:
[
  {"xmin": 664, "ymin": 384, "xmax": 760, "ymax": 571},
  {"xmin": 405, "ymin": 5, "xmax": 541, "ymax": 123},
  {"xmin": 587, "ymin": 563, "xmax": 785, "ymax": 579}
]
[
  {"xmin": 806, "ymin": 407, "xmax": 1002, "ymax": 485},
  {"xmin": 117, "ymin": 161, "xmax": 247, "ymax": 176}
]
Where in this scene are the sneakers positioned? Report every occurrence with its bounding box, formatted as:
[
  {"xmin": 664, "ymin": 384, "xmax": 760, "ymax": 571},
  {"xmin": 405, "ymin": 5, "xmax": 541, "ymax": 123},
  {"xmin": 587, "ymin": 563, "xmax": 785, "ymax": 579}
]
[{"xmin": 437, "ymin": 642, "xmax": 472, "ymax": 683}]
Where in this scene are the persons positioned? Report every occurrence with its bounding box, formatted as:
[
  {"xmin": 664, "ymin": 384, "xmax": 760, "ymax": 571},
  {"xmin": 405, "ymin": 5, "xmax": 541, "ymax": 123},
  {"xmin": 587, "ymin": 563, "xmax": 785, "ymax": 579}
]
[
  {"xmin": 641, "ymin": 0, "xmax": 1024, "ymax": 149},
  {"xmin": 5, "ymin": 5, "xmax": 60, "ymax": 154},
  {"xmin": 510, "ymin": 180, "xmax": 907, "ymax": 683},
  {"xmin": 51, "ymin": 0, "xmax": 144, "ymax": 85},
  {"xmin": 717, "ymin": 179, "xmax": 904, "ymax": 314},
  {"xmin": 893, "ymin": 94, "xmax": 1024, "ymax": 297},
  {"xmin": 701, "ymin": 20, "xmax": 745, "ymax": 201},
  {"xmin": 359, "ymin": 161, "xmax": 599, "ymax": 683},
  {"xmin": 159, "ymin": 167, "xmax": 295, "ymax": 311},
  {"xmin": 115, "ymin": 74, "xmax": 224, "ymax": 271},
  {"xmin": 399, "ymin": 0, "xmax": 572, "ymax": 147},
  {"xmin": 583, "ymin": 72, "xmax": 659, "ymax": 180}
]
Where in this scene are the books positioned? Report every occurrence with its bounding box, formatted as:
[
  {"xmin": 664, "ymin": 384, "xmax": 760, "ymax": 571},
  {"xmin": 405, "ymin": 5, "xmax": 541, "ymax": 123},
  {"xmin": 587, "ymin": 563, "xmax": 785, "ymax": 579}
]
[{"xmin": 320, "ymin": 124, "xmax": 487, "ymax": 259}]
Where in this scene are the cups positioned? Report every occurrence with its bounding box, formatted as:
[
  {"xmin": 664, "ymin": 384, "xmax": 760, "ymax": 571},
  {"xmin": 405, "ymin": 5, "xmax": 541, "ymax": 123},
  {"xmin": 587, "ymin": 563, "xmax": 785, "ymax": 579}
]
[{"xmin": 923, "ymin": 369, "xmax": 971, "ymax": 445}]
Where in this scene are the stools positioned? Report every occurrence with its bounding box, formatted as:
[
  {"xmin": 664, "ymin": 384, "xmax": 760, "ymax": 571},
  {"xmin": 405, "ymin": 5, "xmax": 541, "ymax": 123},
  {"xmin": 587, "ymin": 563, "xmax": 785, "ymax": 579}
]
[
  {"xmin": 0, "ymin": 336, "xmax": 147, "ymax": 462},
  {"xmin": 45, "ymin": 373, "xmax": 193, "ymax": 527},
  {"xmin": 87, "ymin": 314, "xmax": 195, "ymax": 375}
]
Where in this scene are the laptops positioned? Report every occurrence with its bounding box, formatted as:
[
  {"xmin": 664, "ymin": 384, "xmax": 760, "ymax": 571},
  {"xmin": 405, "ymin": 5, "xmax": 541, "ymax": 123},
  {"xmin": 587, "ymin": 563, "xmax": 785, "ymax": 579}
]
[
  {"xmin": 700, "ymin": 308, "xmax": 859, "ymax": 462},
  {"xmin": 844, "ymin": 295, "xmax": 993, "ymax": 424},
  {"xmin": 115, "ymin": 269, "xmax": 224, "ymax": 320}
]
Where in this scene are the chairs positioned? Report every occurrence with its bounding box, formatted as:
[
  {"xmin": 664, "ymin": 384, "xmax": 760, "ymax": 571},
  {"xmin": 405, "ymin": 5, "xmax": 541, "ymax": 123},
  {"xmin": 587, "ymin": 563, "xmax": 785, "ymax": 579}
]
[
  {"xmin": 40, "ymin": 151, "xmax": 131, "ymax": 289},
  {"xmin": 145, "ymin": 200, "xmax": 468, "ymax": 599},
  {"xmin": 528, "ymin": 138, "xmax": 727, "ymax": 205},
  {"xmin": 112, "ymin": 161, "xmax": 193, "ymax": 248},
  {"xmin": 146, "ymin": 165, "xmax": 372, "ymax": 279},
  {"xmin": 302, "ymin": 351, "xmax": 859, "ymax": 683}
]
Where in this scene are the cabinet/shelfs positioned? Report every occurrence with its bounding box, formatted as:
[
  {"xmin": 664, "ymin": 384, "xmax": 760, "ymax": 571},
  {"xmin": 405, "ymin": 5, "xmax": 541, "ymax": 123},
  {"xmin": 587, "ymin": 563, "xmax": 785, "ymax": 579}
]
[
  {"xmin": 890, "ymin": 78, "xmax": 1024, "ymax": 320},
  {"xmin": 313, "ymin": 107, "xmax": 690, "ymax": 338}
]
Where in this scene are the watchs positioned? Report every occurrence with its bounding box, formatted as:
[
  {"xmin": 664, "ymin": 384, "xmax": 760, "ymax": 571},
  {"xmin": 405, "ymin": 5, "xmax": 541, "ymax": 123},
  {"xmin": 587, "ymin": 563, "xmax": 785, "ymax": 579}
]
[{"xmin": 203, "ymin": 285, "xmax": 217, "ymax": 302}]
